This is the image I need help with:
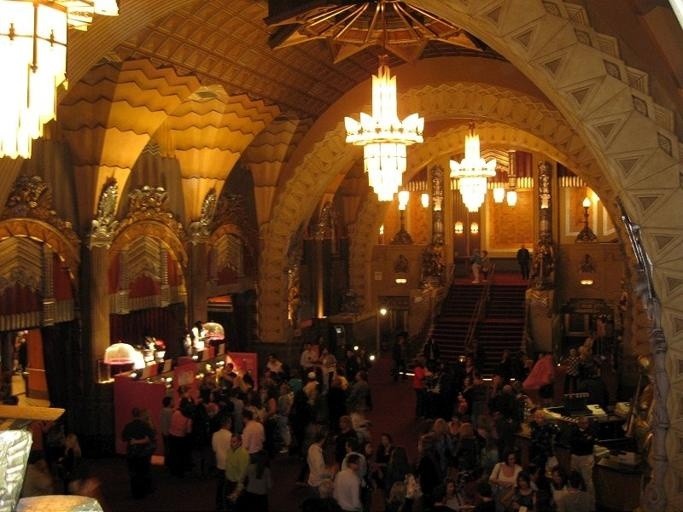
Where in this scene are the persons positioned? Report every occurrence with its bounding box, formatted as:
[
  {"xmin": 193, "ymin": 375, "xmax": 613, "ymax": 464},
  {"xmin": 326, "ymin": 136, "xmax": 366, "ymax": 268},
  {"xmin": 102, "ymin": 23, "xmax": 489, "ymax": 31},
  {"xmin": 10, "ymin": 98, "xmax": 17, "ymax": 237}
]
[
  {"xmin": 469, "ymin": 247, "xmax": 480, "ymax": 283},
  {"xmin": 479, "ymin": 250, "xmax": 489, "ymax": 280},
  {"xmin": 12, "ymin": 329, "xmax": 28, "ymax": 371},
  {"xmin": 53, "ymin": 313, "xmax": 658, "ymax": 511},
  {"xmin": 515, "ymin": 243, "xmax": 530, "ymax": 281}
]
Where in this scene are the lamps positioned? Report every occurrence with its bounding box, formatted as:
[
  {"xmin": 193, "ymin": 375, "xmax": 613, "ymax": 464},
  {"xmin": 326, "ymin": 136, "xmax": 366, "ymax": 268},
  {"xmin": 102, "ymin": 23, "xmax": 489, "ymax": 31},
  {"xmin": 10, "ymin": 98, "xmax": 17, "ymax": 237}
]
[
  {"xmin": 397, "ymin": 203, "xmax": 406, "ymax": 230},
  {"xmin": 343, "ymin": 45, "xmax": 425, "ymax": 203},
  {"xmin": 582, "ymin": 197, "xmax": 591, "ymax": 228},
  {"xmin": 448, "ymin": 120, "xmax": 496, "ymax": 213}
]
